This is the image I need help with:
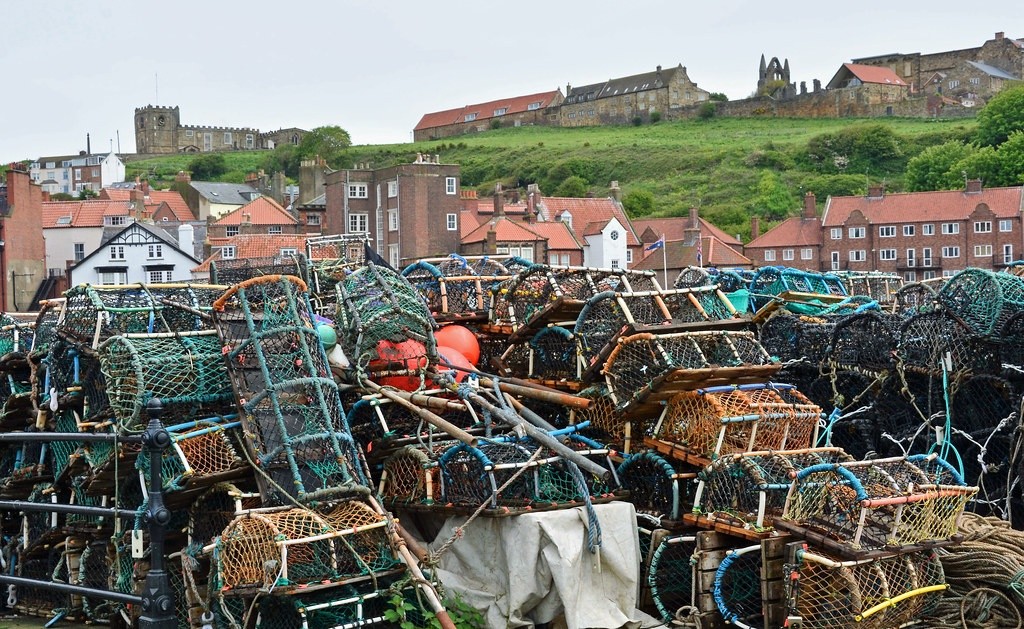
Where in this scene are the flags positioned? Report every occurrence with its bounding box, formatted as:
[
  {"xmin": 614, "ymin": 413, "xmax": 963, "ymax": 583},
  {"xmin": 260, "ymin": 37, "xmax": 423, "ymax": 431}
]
[
  {"xmin": 697, "ymin": 241, "xmax": 702, "ymax": 261},
  {"xmin": 644, "ymin": 235, "xmax": 663, "ymax": 250}
]
